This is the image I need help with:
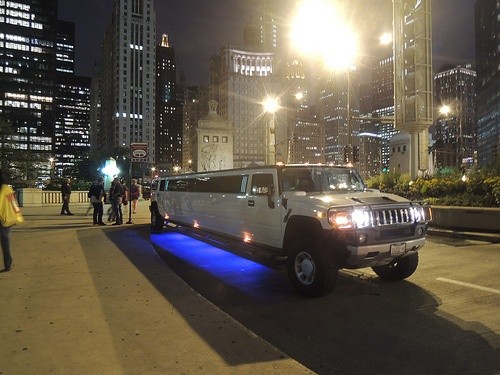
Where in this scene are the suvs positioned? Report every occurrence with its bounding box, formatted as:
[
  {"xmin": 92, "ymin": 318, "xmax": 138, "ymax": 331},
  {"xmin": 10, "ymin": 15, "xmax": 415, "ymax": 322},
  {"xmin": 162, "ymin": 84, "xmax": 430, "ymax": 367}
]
[{"xmin": 149, "ymin": 164, "xmax": 433, "ymax": 297}]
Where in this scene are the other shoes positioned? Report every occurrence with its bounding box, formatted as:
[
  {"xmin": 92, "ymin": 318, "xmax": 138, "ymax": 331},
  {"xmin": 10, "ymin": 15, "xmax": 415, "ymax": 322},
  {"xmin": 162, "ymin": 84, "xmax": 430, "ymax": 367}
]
[
  {"xmin": 94, "ymin": 222, "xmax": 98, "ymax": 224},
  {"xmin": 112, "ymin": 223, "xmax": 120, "ymax": 225},
  {"xmin": 68, "ymin": 213, "xmax": 73, "ymax": 215},
  {"xmin": 126, "ymin": 222, "xmax": 132, "ymax": 223},
  {"xmin": 5, "ymin": 265, "xmax": 14, "ymax": 271},
  {"xmin": 61, "ymin": 213, "xmax": 66, "ymax": 215},
  {"xmin": 98, "ymin": 222, "xmax": 106, "ymax": 225}
]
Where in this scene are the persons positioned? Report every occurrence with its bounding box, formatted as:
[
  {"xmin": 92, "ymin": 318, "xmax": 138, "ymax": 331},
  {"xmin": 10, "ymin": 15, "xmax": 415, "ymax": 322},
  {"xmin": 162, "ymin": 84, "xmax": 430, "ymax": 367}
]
[
  {"xmin": 88, "ymin": 177, "xmax": 107, "ymax": 225},
  {"xmin": 111, "ymin": 178, "xmax": 125, "ymax": 225},
  {"xmin": 107, "ymin": 180, "xmax": 117, "ymax": 222},
  {"xmin": 60, "ymin": 178, "xmax": 74, "ymax": 216},
  {"xmin": 128, "ymin": 179, "xmax": 141, "ymax": 213},
  {"xmin": 0, "ymin": 172, "xmax": 24, "ymax": 272}
]
